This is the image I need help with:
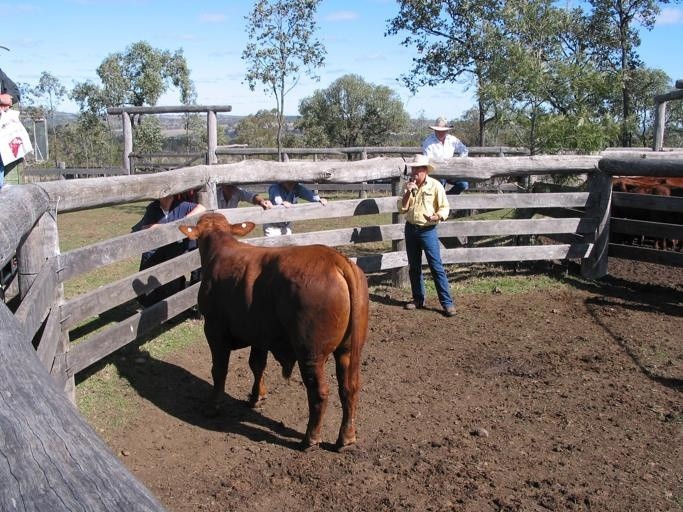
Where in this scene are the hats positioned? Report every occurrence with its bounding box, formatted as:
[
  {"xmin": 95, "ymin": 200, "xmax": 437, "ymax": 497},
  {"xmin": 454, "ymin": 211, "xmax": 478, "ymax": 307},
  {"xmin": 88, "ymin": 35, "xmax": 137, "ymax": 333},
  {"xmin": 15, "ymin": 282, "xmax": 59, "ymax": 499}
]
[
  {"xmin": 405, "ymin": 154, "xmax": 434, "ymax": 172},
  {"xmin": 427, "ymin": 117, "xmax": 453, "ymax": 131}
]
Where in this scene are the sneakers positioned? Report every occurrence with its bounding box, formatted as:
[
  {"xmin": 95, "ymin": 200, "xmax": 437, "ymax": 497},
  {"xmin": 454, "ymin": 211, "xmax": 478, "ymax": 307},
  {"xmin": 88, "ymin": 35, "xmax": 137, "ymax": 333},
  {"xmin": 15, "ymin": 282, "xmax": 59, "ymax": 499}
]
[
  {"xmin": 404, "ymin": 300, "xmax": 424, "ymax": 309},
  {"xmin": 445, "ymin": 306, "xmax": 456, "ymax": 316}
]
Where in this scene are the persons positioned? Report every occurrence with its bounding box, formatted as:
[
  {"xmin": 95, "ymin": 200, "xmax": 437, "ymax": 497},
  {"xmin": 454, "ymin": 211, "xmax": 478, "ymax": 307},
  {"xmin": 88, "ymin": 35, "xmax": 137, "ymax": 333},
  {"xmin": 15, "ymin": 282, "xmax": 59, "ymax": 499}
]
[
  {"xmin": 129, "ymin": 186, "xmax": 208, "ymax": 319},
  {"xmin": 22, "ymin": 47, "xmax": 683, "ymax": 197},
  {"xmin": 186, "ymin": 183, "xmax": 274, "ymax": 318},
  {"xmin": 263, "ymin": 179, "xmax": 326, "ymax": 239},
  {"xmin": 422, "ymin": 117, "xmax": 469, "ymax": 194},
  {"xmin": 397, "ymin": 152, "xmax": 456, "ymax": 316}
]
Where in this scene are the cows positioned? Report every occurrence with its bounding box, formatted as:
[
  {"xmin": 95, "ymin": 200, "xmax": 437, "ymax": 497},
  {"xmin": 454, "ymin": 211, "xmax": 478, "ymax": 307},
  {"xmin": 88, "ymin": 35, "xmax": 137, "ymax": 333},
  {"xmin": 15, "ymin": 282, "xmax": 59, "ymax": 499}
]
[
  {"xmin": 177, "ymin": 211, "xmax": 369, "ymax": 454},
  {"xmin": 611, "ymin": 176, "xmax": 683, "ymax": 251}
]
